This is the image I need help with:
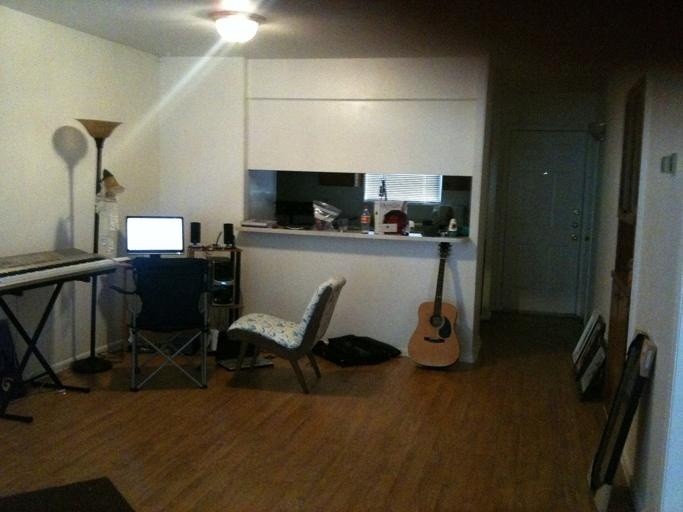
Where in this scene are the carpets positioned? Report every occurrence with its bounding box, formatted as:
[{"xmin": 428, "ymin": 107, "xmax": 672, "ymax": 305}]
[{"xmin": 0, "ymin": 476, "xmax": 132, "ymax": 512}]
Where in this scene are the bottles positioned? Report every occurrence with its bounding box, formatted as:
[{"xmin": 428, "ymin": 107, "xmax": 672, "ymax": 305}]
[
  {"xmin": 448, "ymin": 218, "xmax": 458, "ymax": 238},
  {"xmin": 360, "ymin": 206, "xmax": 372, "ymax": 233}
]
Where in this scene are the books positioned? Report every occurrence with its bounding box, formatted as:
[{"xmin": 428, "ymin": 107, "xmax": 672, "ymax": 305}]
[
  {"xmin": 207, "ymin": 328, "xmax": 219, "ymax": 353},
  {"xmin": 111, "ymin": 256, "xmax": 132, "ymax": 262},
  {"xmin": 215, "ymin": 356, "xmax": 273, "ymax": 373},
  {"xmin": 373, "ymin": 199, "xmax": 408, "ymax": 233},
  {"xmin": 241, "ymin": 218, "xmax": 277, "ymax": 228}
]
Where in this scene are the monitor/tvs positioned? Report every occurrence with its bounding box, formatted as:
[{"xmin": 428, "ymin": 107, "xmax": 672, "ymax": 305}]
[{"xmin": 125, "ymin": 215, "xmax": 185, "ymax": 258}]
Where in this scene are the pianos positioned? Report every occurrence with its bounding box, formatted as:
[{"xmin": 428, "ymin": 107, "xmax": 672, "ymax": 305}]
[{"xmin": 0, "ymin": 249, "xmax": 119, "ymax": 295}]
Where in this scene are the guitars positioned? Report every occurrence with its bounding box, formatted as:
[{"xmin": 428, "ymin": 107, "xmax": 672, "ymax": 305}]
[{"xmin": 408, "ymin": 242, "xmax": 460, "ymax": 369}]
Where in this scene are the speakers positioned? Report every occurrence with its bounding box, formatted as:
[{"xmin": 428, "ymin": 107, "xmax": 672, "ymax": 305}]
[
  {"xmin": 189, "ymin": 222, "xmax": 201, "ymax": 245},
  {"xmin": 223, "ymin": 222, "xmax": 234, "ymax": 245}
]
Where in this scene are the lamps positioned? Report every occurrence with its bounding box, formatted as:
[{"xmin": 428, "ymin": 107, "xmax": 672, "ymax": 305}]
[
  {"xmin": 70, "ymin": 118, "xmax": 121, "ymax": 374},
  {"xmin": 208, "ymin": 8, "xmax": 268, "ymax": 44}
]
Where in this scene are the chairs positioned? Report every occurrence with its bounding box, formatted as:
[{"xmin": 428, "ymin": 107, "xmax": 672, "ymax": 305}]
[
  {"xmin": 121, "ymin": 259, "xmax": 214, "ymax": 391},
  {"xmin": 230, "ymin": 272, "xmax": 347, "ymax": 386}
]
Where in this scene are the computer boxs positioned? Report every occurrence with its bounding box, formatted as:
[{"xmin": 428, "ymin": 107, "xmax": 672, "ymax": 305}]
[{"xmin": 207, "ymin": 255, "xmax": 234, "ymax": 307}]
